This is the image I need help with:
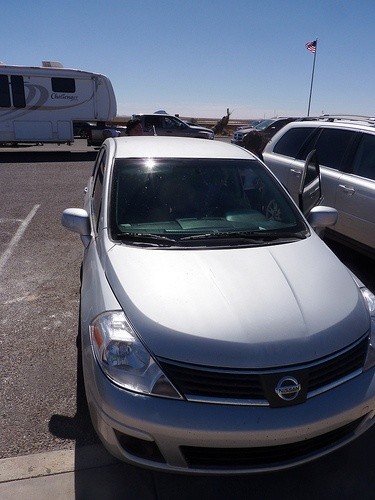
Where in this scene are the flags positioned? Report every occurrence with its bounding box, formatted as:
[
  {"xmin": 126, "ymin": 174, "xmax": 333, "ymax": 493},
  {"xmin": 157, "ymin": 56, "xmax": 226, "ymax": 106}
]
[{"xmin": 305, "ymin": 40, "xmax": 316, "ymax": 53}]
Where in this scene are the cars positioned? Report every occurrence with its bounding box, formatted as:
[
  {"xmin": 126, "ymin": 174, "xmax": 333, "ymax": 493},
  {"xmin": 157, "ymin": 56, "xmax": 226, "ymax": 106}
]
[
  {"xmin": 261, "ymin": 119, "xmax": 375, "ymax": 273},
  {"xmin": 123, "ymin": 113, "xmax": 215, "ymax": 140},
  {"xmin": 60, "ymin": 133, "xmax": 375, "ymax": 478},
  {"xmin": 231, "ymin": 115, "xmax": 320, "ymax": 159}
]
